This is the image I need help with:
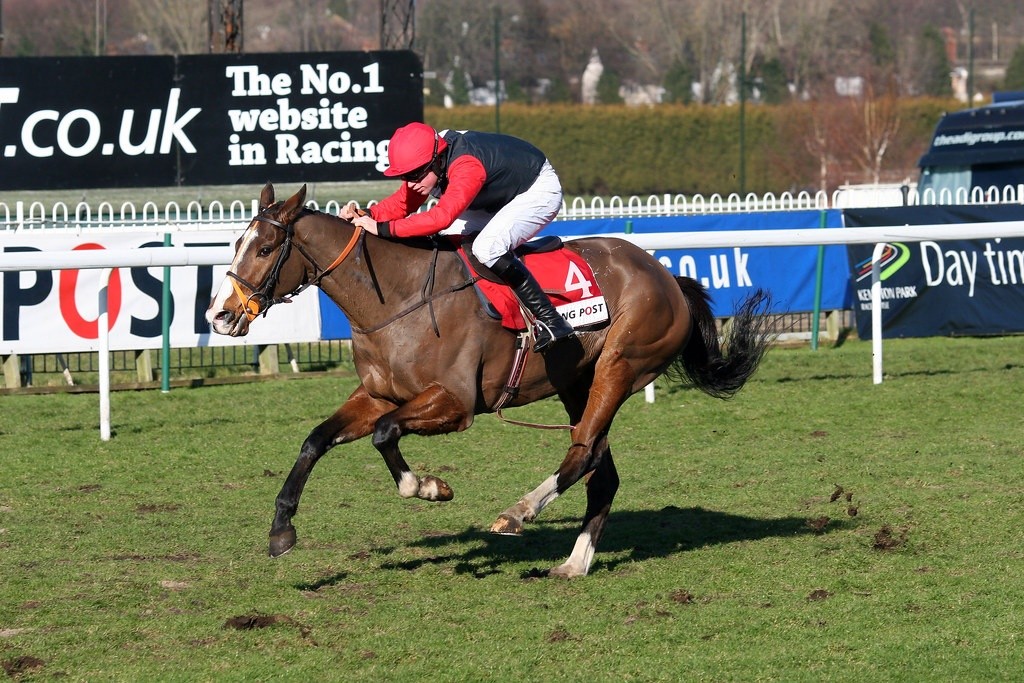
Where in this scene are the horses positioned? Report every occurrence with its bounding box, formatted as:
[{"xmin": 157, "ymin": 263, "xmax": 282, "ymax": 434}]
[{"xmin": 203, "ymin": 177, "xmax": 809, "ymax": 581}]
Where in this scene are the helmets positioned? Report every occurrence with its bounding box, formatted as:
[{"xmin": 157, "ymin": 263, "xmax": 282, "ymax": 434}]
[{"xmin": 382, "ymin": 122, "xmax": 448, "ymax": 179}]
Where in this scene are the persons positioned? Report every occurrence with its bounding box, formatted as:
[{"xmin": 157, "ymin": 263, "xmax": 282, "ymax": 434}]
[{"xmin": 338, "ymin": 121, "xmax": 574, "ymax": 354}]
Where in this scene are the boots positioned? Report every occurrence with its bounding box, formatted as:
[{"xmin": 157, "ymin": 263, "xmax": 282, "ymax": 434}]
[{"xmin": 492, "ymin": 250, "xmax": 572, "ymax": 351}]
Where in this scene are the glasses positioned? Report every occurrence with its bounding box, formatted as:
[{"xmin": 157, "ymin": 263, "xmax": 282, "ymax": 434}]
[{"xmin": 400, "ymin": 130, "xmax": 437, "ymax": 183}]
